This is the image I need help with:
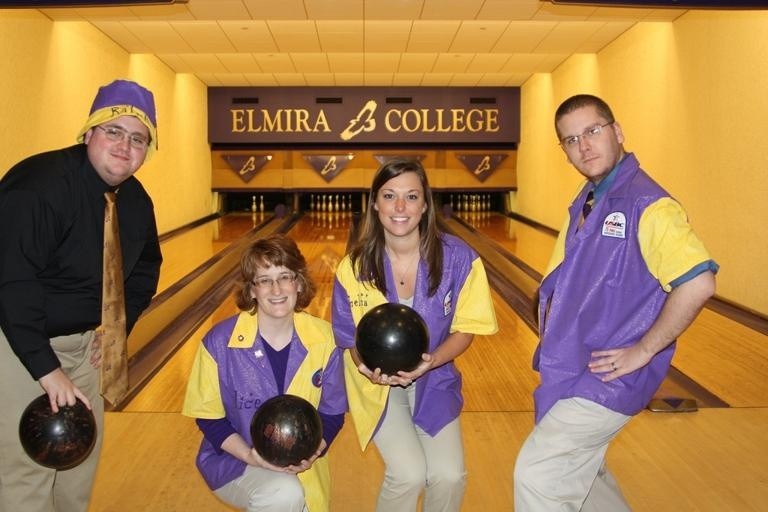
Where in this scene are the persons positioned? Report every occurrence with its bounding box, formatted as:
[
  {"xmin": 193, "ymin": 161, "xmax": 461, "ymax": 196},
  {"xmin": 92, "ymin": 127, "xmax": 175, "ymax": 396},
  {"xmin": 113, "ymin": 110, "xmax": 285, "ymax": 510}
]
[
  {"xmin": 510, "ymin": 93, "xmax": 720, "ymax": 512},
  {"xmin": 179, "ymin": 235, "xmax": 349, "ymax": 512},
  {"xmin": 0, "ymin": 76, "xmax": 163, "ymax": 511},
  {"xmin": 330, "ymin": 155, "xmax": 501, "ymax": 511}
]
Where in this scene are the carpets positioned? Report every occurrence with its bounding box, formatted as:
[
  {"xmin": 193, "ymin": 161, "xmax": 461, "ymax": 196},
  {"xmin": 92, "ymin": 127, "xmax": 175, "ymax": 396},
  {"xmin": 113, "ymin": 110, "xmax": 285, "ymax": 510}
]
[
  {"xmin": 576, "ymin": 189, "xmax": 596, "ymax": 235},
  {"xmin": 98, "ymin": 187, "xmax": 134, "ymax": 409}
]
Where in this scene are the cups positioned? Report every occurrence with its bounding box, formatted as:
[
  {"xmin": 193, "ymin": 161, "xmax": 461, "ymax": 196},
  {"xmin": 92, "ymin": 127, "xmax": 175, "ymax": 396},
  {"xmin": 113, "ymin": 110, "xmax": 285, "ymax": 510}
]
[
  {"xmin": 558, "ymin": 120, "xmax": 614, "ymax": 147},
  {"xmin": 246, "ymin": 270, "xmax": 300, "ymax": 290},
  {"xmin": 96, "ymin": 125, "xmax": 151, "ymax": 149}
]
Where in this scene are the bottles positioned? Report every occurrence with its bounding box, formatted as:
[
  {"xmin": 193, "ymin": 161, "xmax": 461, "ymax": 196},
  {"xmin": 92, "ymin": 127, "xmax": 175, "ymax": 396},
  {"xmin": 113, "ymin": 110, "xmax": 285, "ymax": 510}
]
[{"xmin": 79, "ymin": 79, "xmax": 160, "ymax": 154}]
